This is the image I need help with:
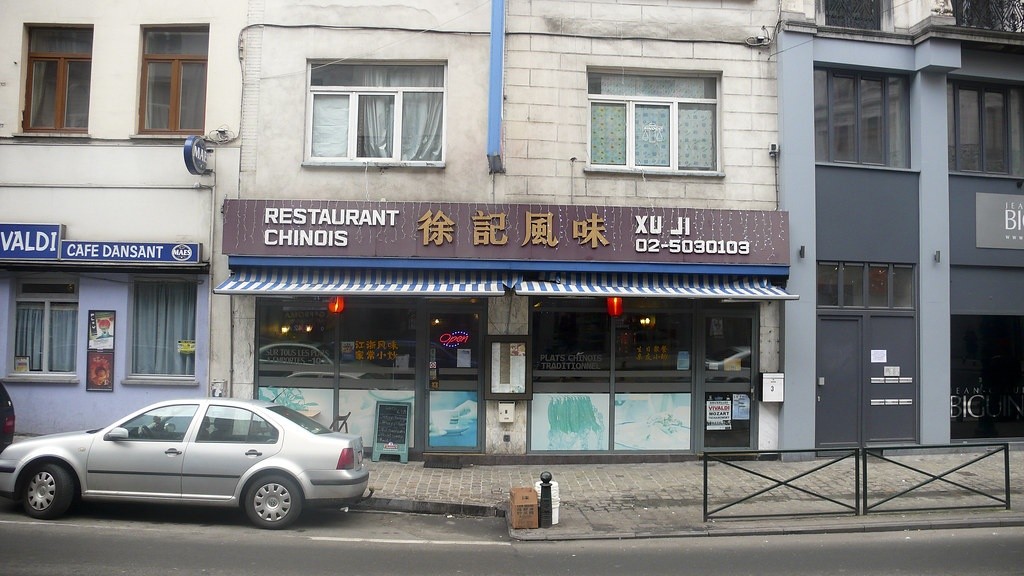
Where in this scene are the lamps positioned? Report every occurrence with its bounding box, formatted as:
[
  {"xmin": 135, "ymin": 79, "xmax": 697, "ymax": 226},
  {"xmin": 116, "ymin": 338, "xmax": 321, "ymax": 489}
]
[
  {"xmin": 934, "ymin": 250, "xmax": 940, "ymax": 262},
  {"xmin": 800, "ymin": 246, "xmax": 805, "ymax": 259}
]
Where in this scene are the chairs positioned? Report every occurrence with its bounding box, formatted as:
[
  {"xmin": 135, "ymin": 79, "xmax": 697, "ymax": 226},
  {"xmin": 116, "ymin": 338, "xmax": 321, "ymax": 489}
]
[
  {"xmin": 210, "ymin": 415, "xmax": 232, "ymax": 441},
  {"xmin": 329, "ymin": 411, "xmax": 351, "ymax": 433},
  {"xmin": 196, "ymin": 416, "xmax": 211, "ymax": 440}
]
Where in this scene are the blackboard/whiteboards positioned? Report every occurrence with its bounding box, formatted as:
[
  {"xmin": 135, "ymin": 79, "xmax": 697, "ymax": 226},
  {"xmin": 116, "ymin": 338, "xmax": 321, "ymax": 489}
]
[{"xmin": 377, "ymin": 403, "xmax": 408, "ymax": 445}]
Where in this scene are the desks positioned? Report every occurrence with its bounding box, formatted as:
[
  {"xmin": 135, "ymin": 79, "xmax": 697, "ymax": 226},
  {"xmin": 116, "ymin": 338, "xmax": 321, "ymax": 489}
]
[{"xmin": 298, "ymin": 410, "xmax": 321, "ymax": 422}]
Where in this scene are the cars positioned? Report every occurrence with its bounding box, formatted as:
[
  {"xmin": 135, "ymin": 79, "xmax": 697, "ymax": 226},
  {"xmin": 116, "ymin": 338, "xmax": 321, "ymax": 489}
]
[{"xmin": 0, "ymin": 395, "xmax": 370, "ymax": 530}]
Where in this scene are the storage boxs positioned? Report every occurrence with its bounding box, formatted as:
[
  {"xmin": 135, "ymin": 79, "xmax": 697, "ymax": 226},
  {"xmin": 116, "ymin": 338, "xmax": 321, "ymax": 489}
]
[
  {"xmin": 510, "ymin": 486, "xmax": 539, "ymax": 530},
  {"xmin": 720, "ymin": 358, "xmax": 741, "ymax": 371}
]
